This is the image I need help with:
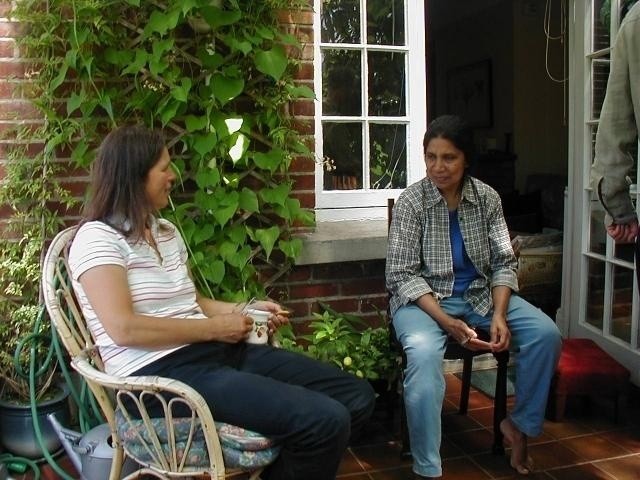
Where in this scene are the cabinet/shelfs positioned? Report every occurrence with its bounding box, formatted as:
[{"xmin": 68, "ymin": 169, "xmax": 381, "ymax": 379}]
[{"xmin": 473, "ymin": 152, "xmax": 518, "ymax": 189}]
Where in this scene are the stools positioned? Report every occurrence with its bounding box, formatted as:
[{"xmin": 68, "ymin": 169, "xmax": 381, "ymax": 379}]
[{"xmin": 545, "ymin": 336, "xmax": 632, "ymax": 425}]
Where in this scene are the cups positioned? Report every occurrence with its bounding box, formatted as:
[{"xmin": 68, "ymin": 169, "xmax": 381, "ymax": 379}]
[{"xmin": 241, "ymin": 309, "xmax": 270, "ymax": 345}]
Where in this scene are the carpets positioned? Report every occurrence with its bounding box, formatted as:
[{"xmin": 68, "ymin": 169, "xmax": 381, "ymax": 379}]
[{"xmin": 452, "ymin": 362, "xmax": 517, "ymax": 398}]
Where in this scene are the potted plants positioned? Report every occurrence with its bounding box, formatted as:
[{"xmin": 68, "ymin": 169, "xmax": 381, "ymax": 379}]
[{"xmin": 0, "ymin": 0, "xmax": 74, "ymax": 460}]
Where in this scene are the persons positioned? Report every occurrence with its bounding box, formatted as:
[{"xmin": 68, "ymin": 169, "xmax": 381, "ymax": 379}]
[
  {"xmin": 590, "ymin": 0, "xmax": 640, "ymax": 289},
  {"xmin": 384, "ymin": 115, "xmax": 563, "ymax": 480},
  {"xmin": 67, "ymin": 125, "xmax": 377, "ymax": 480}
]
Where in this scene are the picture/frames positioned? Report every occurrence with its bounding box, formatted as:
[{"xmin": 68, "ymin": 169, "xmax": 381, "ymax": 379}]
[{"xmin": 444, "ymin": 57, "xmax": 495, "ymax": 130}]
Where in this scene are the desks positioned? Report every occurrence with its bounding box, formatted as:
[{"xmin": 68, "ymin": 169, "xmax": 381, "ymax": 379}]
[{"xmin": 507, "ymin": 228, "xmax": 564, "ymax": 294}]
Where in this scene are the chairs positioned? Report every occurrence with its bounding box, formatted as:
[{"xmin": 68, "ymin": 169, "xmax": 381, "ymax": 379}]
[
  {"xmin": 38, "ymin": 225, "xmax": 288, "ymax": 480},
  {"xmin": 385, "ymin": 195, "xmax": 509, "ymax": 466}
]
